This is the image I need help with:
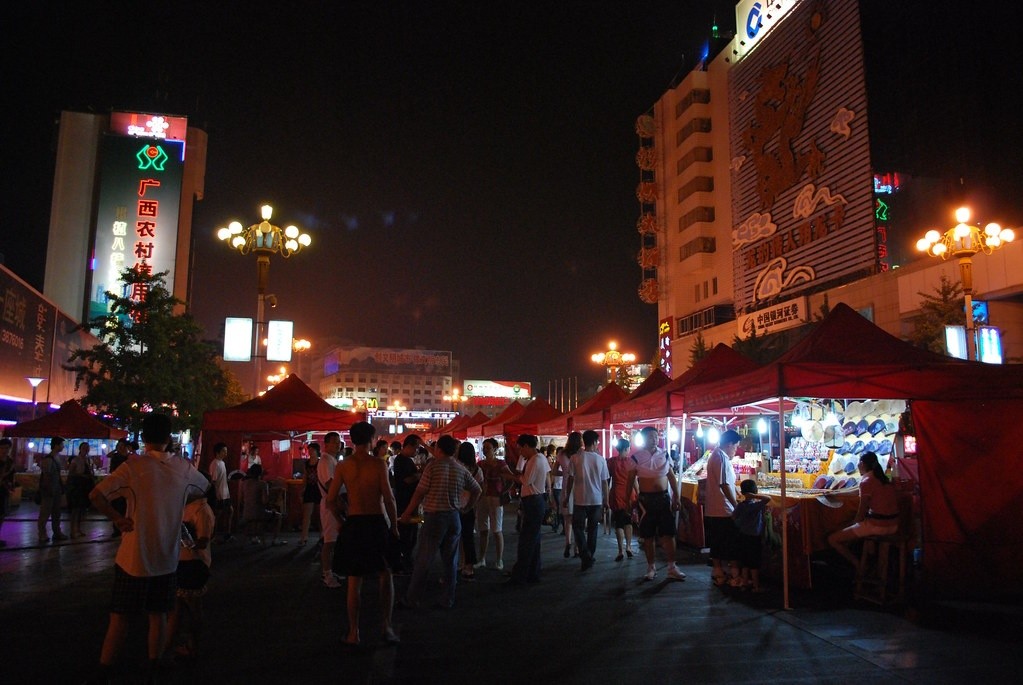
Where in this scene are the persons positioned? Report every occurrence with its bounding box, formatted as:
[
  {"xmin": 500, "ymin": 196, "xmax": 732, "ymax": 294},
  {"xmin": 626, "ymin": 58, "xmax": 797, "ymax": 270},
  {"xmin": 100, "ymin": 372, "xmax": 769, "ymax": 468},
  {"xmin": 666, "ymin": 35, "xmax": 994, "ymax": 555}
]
[
  {"xmin": 704, "ymin": 429, "xmax": 742, "ymax": 585},
  {"xmin": 624, "ymin": 427, "xmax": 686, "ymax": 580},
  {"xmin": 497, "ymin": 433, "xmax": 552, "ymax": 579},
  {"xmin": 538, "ymin": 444, "xmax": 566, "ymax": 535},
  {"xmin": 209, "ymin": 442, "xmax": 239, "ymax": 545},
  {"xmin": 86, "ymin": 414, "xmax": 215, "ymax": 685},
  {"xmin": 66, "ymin": 442, "xmax": 94, "ymax": 538},
  {"xmin": 607, "ymin": 439, "xmax": 639, "ymax": 561},
  {"xmin": 38, "ymin": 436, "xmax": 68, "ymax": 541},
  {"xmin": 248, "ymin": 446, "xmax": 261, "ymax": 469},
  {"xmin": 563, "ymin": 430, "xmax": 611, "ymax": 570},
  {"xmin": 296, "ymin": 443, "xmax": 321, "ymax": 547},
  {"xmin": 238, "ymin": 464, "xmax": 288, "ymax": 546},
  {"xmin": 327, "ymin": 422, "xmax": 400, "ymax": 647},
  {"xmin": 733, "ymin": 480, "xmax": 771, "ymax": 594},
  {"xmin": 551, "ymin": 431, "xmax": 584, "ymax": 559},
  {"xmin": 473, "ymin": 438, "xmax": 514, "ymax": 570},
  {"xmin": 317, "ymin": 432, "xmax": 347, "ymax": 588},
  {"xmin": 372, "ymin": 433, "xmax": 485, "ymax": 610},
  {"xmin": 824, "ymin": 451, "xmax": 900, "ymax": 566},
  {"xmin": 0, "ymin": 439, "xmax": 16, "ymax": 547}
]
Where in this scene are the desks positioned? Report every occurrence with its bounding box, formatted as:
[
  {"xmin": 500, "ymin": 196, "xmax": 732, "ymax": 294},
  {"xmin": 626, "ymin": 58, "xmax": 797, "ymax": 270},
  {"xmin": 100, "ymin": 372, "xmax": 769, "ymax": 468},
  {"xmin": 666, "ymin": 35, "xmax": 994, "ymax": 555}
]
[
  {"xmin": 677, "ymin": 482, "xmax": 705, "ymax": 548},
  {"xmin": 747, "ymin": 486, "xmax": 861, "ymax": 600}
]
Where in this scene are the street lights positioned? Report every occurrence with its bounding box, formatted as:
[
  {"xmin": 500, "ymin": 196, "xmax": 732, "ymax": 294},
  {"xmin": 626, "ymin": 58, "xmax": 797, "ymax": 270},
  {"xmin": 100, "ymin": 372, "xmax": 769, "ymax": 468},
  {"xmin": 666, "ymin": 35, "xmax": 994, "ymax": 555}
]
[
  {"xmin": 25, "ymin": 376, "xmax": 48, "ymax": 419},
  {"xmin": 913, "ymin": 206, "xmax": 1018, "ymax": 362},
  {"xmin": 217, "ymin": 204, "xmax": 314, "ymax": 400},
  {"xmin": 291, "ymin": 339, "xmax": 312, "ymax": 381},
  {"xmin": 591, "ymin": 339, "xmax": 636, "ymax": 388},
  {"xmin": 386, "ymin": 400, "xmax": 407, "ymax": 440},
  {"xmin": 442, "ymin": 387, "xmax": 469, "ymax": 413}
]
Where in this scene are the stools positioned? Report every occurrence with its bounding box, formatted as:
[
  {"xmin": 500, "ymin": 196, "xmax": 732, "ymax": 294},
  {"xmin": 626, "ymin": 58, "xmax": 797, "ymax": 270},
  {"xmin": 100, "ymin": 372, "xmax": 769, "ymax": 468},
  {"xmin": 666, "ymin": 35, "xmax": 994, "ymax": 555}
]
[{"xmin": 859, "ymin": 535, "xmax": 913, "ymax": 609}]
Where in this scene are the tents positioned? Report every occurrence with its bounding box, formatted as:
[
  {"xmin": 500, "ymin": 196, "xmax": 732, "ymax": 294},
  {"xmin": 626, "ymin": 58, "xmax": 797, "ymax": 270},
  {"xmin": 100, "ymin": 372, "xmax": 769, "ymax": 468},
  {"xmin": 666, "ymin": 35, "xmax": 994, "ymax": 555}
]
[
  {"xmin": 683, "ymin": 303, "xmax": 1023, "ymax": 573},
  {"xmin": 202, "ymin": 373, "xmax": 363, "ymax": 439},
  {"xmin": 432, "ymin": 302, "xmax": 758, "ymax": 459},
  {"xmin": 3, "ymin": 399, "xmax": 128, "ymax": 441}
]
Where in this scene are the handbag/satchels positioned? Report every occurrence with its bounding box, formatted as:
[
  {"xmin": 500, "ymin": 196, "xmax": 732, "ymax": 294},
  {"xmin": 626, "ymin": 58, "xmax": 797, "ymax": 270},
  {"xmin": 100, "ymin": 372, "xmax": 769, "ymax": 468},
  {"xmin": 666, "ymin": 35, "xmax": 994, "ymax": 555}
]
[{"xmin": 87, "ymin": 478, "xmax": 96, "ymax": 493}]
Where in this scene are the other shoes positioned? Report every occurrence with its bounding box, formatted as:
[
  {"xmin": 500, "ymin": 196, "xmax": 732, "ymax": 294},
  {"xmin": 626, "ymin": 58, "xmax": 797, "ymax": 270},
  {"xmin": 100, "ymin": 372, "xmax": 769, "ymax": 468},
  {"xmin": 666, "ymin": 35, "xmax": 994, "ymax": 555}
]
[
  {"xmin": 668, "ymin": 570, "xmax": 686, "ymax": 580},
  {"xmin": 580, "ymin": 552, "xmax": 594, "ymax": 570},
  {"xmin": 71, "ymin": 532, "xmax": 85, "ymax": 539},
  {"xmin": 643, "ymin": 568, "xmax": 657, "ymax": 580},
  {"xmin": 574, "ymin": 552, "xmax": 579, "ymax": 557},
  {"xmin": 343, "ymin": 634, "xmax": 360, "ymax": 645},
  {"xmin": 627, "ymin": 549, "xmax": 633, "ymax": 557},
  {"xmin": 461, "ymin": 563, "xmax": 475, "ymax": 576},
  {"xmin": 392, "ymin": 564, "xmax": 415, "ymax": 576},
  {"xmin": 472, "ymin": 561, "xmax": 486, "ymax": 569},
  {"xmin": 503, "ymin": 575, "xmax": 524, "ymax": 586},
  {"xmin": 564, "ymin": 545, "xmax": 571, "ymax": 558},
  {"xmin": 40, "ymin": 536, "xmax": 50, "ymax": 542},
  {"xmin": 711, "ymin": 569, "xmax": 771, "ymax": 593},
  {"xmin": 210, "ymin": 532, "xmax": 326, "ymax": 548},
  {"xmin": 382, "ymin": 630, "xmax": 401, "ymax": 642},
  {"xmin": 320, "ymin": 576, "xmax": 341, "ymax": 589},
  {"xmin": 494, "ymin": 560, "xmax": 504, "ymax": 570},
  {"xmin": 175, "ymin": 643, "xmax": 199, "ymax": 657},
  {"xmin": 858, "ymin": 567, "xmax": 876, "ymax": 580},
  {"xmin": 52, "ymin": 533, "xmax": 68, "ymax": 540},
  {"xmin": 616, "ymin": 554, "xmax": 623, "ymax": 561}
]
